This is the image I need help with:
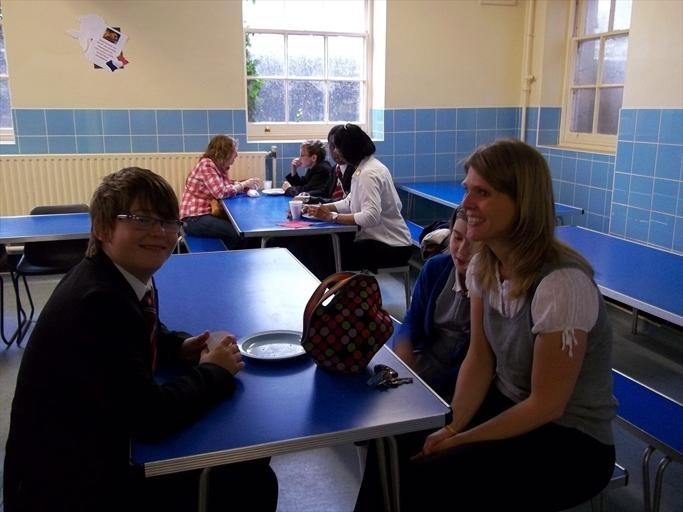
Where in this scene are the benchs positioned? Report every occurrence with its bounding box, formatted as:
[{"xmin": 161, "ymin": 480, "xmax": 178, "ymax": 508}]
[{"xmin": 612, "ymin": 368, "xmax": 683, "ymax": 510}]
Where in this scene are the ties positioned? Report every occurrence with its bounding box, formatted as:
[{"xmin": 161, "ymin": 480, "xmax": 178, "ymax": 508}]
[
  {"xmin": 144, "ymin": 288, "xmax": 161, "ymax": 371},
  {"xmin": 330, "ymin": 167, "xmax": 345, "ymax": 202}
]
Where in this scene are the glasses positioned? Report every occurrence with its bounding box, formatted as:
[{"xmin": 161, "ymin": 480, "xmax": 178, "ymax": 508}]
[{"xmin": 115, "ymin": 214, "xmax": 183, "ymax": 235}]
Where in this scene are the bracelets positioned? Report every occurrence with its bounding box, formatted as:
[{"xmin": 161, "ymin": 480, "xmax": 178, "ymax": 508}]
[
  {"xmin": 329, "ymin": 212, "xmax": 339, "ymax": 223},
  {"xmin": 443, "ymin": 425, "xmax": 456, "ymax": 436},
  {"xmin": 239, "ymin": 183, "xmax": 244, "ymax": 191}
]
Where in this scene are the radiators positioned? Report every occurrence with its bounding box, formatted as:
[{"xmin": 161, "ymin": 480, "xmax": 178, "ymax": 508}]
[{"xmin": 0, "ymin": 145, "xmax": 277, "ymax": 215}]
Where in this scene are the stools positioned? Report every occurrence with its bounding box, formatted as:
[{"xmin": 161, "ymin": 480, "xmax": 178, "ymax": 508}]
[
  {"xmin": 401, "ymin": 216, "xmax": 423, "ymax": 249},
  {"xmin": 588, "ymin": 465, "xmax": 627, "ymax": 512},
  {"xmin": 181, "ymin": 222, "xmax": 227, "ymax": 253},
  {"xmin": 364, "ymin": 264, "xmax": 412, "ymax": 320}
]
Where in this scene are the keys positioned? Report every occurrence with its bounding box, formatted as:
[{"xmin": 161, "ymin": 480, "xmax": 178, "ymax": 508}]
[{"xmin": 367, "ymin": 365, "xmax": 413, "ymax": 391}]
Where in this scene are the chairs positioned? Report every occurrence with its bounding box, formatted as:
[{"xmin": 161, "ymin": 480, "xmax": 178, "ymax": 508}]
[{"xmin": 14, "ymin": 203, "xmax": 90, "ymax": 343}]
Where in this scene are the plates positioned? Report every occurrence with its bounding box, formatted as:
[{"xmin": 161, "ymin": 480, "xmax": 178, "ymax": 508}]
[
  {"xmin": 237, "ymin": 330, "xmax": 306, "ymax": 359},
  {"xmin": 301, "ymin": 211, "xmax": 337, "ymax": 219},
  {"xmin": 262, "ymin": 189, "xmax": 286, "ymax": 194}
]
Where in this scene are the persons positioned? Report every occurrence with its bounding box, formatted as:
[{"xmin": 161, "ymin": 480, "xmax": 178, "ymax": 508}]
[
  {"xmin": 4, "ymin": 166, "xmax": 278, "ymax": 512},
  {"xmin": 398, "ymin": 144, "xmax": 619, "ymax": 512},
  {"xmin": 282, "ymin": 139, "xmax": 333, "ymax": 197},
  {"xmin": 287, "ymin": 124, "xmax": 414, "ymax": 272},
  {"xmin": 178, "ymin": 135, "xmax": 260, "ymax": 250},
  {"xmin": 353, "ymin": 206, "xmax": 480, "ymax": 512},
  {"xmin": 293, "ymin": 126, "xmax": 355, "ymax": 204}
]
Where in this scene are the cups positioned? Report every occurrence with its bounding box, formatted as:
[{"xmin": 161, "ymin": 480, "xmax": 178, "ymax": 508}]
[
  {"xmin": 262, "ymin": 180, "xmax": 271, "ymax": 188},
  {"xmin": 289, "ymin": 200, "xmax": 302, "ymax": 219}
]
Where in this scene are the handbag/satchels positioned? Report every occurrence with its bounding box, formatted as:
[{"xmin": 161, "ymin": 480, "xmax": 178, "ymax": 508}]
[{"xmin": 299, "ymin": 270, "xmax": 396, "ymax": 383}]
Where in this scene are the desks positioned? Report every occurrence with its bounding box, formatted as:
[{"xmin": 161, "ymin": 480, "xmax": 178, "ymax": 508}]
[
  {"xmin": 398, "ymin": 182, "xmax": 584, "ymax": 251},
  {"xmin": 547, "ymin": 225, "xmax": 682, "ymax": 336},
  {"xmin": 222, "ymin": 192, "xmax": 361, "ymax": 274},
  {"xmin": 142, "ymin": 247, "xmax": 452, "ymax": 511},
  {"xmin": 0, "ymin": 212, "xmax": 92, "ymax": 344}
]
